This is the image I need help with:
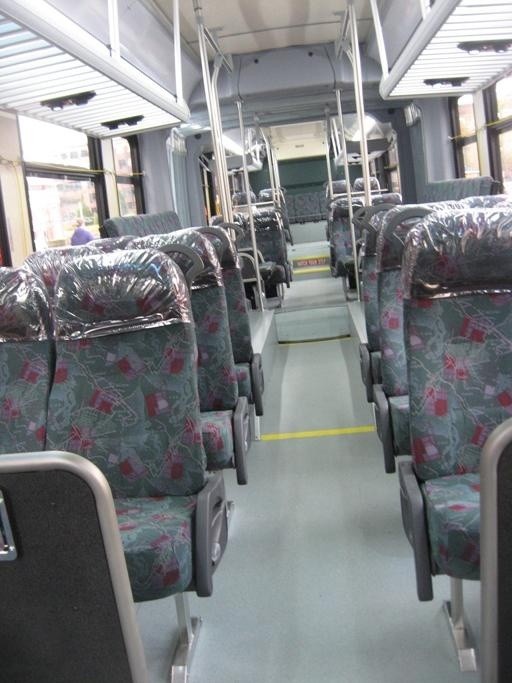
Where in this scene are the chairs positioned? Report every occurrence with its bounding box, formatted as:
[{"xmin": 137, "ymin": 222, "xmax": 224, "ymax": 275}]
[
  {"xmin": 323, "ymin": 168, "xmax": 512, "ymax": 682},
  {"xmin": 2, "ymin": 184, "xmax": 294, "ymax": 683}
]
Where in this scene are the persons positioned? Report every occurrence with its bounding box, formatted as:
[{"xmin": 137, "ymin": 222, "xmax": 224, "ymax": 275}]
[{"xmin": 71, "ymin": 219, "xmax": 94, "ymax": 246}]
